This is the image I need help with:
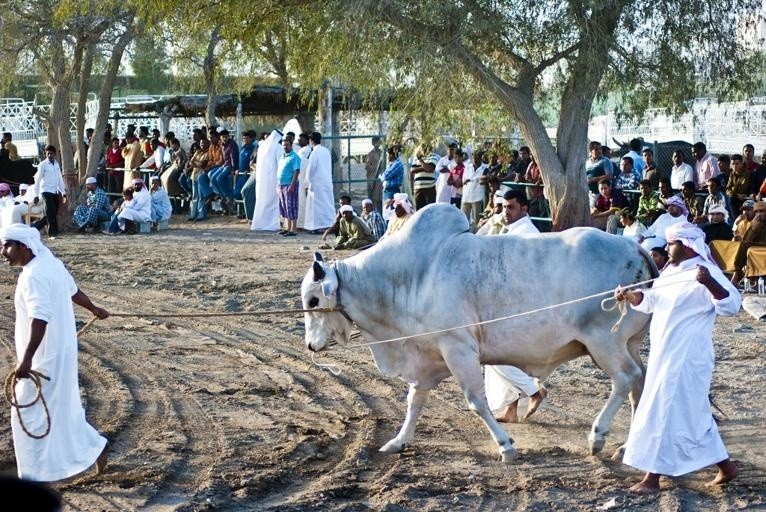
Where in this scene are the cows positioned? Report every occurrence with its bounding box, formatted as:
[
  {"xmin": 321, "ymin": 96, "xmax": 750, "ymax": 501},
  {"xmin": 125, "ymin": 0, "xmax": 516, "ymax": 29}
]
[{"xmin": 300, "ymin": 202, "xmax": 661, "ymax": 463}]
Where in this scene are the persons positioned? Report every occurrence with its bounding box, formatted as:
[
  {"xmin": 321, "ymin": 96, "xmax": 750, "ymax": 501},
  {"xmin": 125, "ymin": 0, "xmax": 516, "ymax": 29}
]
[
  {"xmin": 0, "ymin": 473, "xmax": 64, "ymax": 512},
  {"xmin": 363, "ymin": 136, "xmax": 551, "ymax": 231},
  {"xmin": 1, "ymin": 132, "xmax": 35, "ymax": 194},
  {"xmin": 587, "ymin": 139, "xmax": 766, "ymax": 285},
  {"xmin": 481, "ymin": 187, "xmax": 548, "ymax": 424},
  {"xmin": 1, "ymin": 219, "xmax": 111, "ymax": 481},
  {"xmin": 32, "ymin": 145, "xmax": 66, "ymax": 237},
  {"xmin": 614, "ymin": 221, "xmax": 743, "ymax": 496},
  {"xmin": 73, "ymin": 123, "xmax": 238, "ymax": 220},
  {"xmin": 323, "ymin": 194, "xmax": 413, "ymax": 250},
  {"xmin": 73, "ymin": 176, "xmax": 112, "ymax": 234},
  {"xmin": 109, "ymin": 176, "xmax": 172, "ymax": 233},
  {"xmin": 231, "ymin": 128, "xmax": 337, "ymax": 234}
]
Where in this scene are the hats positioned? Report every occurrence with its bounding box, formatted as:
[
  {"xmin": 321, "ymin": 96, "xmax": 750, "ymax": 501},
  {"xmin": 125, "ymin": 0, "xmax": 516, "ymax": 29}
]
[
  {"xmin": 340, "ymin": 205, "xmax": 353, "ymax": 212},
  {"xmin": 86, "ymin": 176, "xmax": 97, "ymax": 184},
  {"xmin": 361, "ymin": 199, "xmax": 372, "ymax": 205},
  {"xmin": 620, "ymin": 207, "xmax": 633, "ymax": 216},
  {"xmin": 740, "ymin": 200, "xmax": 755, "ymax": 210}
]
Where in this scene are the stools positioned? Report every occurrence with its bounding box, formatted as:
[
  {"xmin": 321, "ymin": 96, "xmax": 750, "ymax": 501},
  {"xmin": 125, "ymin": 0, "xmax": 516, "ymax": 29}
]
[
  {"xmin": 22, "ymin": 198, "xmax": 55, "ymax": 235},
  {"xmin": 137, "ymin": 222, "xmax": 150, "ymax": 233},
  {"xmin": 101, "ymin": 221, "xmax": 111, "ymax": 230},
  {"xmin": 157, "ymin": 219, "xmax": 168, "ymax": 230}
]
